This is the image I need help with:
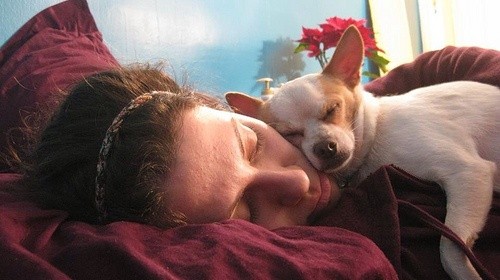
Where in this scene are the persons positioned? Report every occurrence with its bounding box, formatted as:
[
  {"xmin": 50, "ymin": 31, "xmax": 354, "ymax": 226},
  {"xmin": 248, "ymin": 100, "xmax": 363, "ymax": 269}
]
[{"xmin": 21, "ymin": 46, "xmax": 500, "ymax": 279}]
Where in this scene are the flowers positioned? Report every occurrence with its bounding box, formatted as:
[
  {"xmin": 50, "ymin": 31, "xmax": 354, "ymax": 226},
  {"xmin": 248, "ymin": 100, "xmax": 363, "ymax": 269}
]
[{"xmin": 291, "ymin": 16, "xmax": 388, "ymax": 79}]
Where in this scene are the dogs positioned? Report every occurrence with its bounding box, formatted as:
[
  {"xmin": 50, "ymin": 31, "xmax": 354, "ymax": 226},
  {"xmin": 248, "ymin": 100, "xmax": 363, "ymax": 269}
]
[{"xmin": 225, "ymin": 25, "xmax": 500, "ymax": 280}]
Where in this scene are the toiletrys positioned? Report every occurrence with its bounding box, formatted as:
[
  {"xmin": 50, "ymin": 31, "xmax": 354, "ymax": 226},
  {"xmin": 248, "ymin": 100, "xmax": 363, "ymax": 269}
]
[{"xmin": 256, "ymin": 77, "xmax": 273, "ymax": 102}]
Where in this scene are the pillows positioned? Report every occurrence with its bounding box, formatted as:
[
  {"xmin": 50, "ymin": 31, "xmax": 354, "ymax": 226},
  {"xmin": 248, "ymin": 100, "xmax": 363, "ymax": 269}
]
[{"xmin": 0, "ymin": 0, "xmax": 400, "ymax": 280}]
